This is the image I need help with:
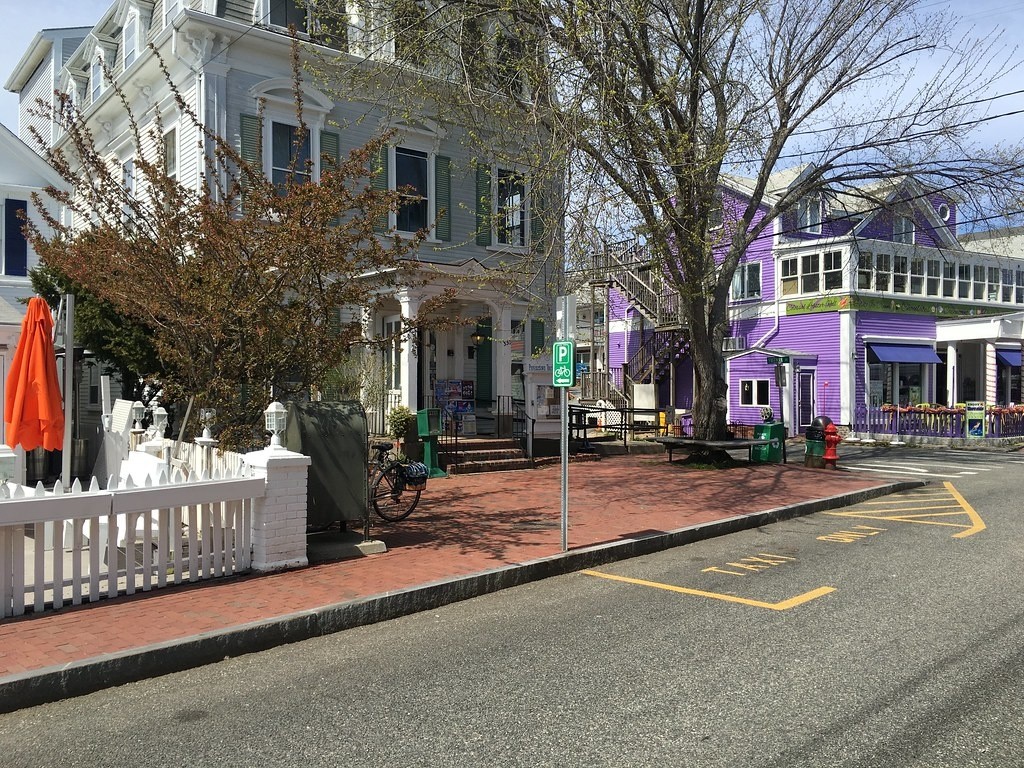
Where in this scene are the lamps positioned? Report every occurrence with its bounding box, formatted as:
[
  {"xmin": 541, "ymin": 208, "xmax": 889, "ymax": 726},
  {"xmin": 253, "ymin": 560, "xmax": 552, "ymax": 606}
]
[
  {"xmin": 132, "ymin": 400, "xmax": 145, "ymax": 422},
  {"xmin": 263, "ymin": 401, "xmax": 288, "ymax": 435},
  {"xmin": 152, "ymin": 407, "xmax": 168, "ymax": 432},
  {"xmin": 200, "ymin": 408, "xmax": 216, "ymax": 429},
  {"xmin": 0, "ymin": 444, "xmax": 16, "ymax": 466},
  {"xmin": 468, "ymin": 330, "xmax": 485, "ymax": 358}
]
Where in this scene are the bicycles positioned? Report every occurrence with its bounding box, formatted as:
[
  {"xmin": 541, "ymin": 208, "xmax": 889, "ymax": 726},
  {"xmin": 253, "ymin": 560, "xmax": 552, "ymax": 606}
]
[{"xmin": 368, "ymin": 442, "xmax": 428, "ymax": 522}]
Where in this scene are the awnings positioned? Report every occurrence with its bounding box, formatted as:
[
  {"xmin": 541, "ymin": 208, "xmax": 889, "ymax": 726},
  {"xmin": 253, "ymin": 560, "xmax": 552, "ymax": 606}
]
[
  {"xmin": 996, "ymin": 352, "xmax": 1022, "ymax": 367},
  {"xmin": 867, "ymin": 343, "xmax": 943, "ymax": 364}
]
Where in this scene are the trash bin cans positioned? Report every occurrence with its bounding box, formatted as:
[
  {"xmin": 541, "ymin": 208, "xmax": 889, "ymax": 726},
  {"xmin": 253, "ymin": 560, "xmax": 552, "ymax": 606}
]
[
  {"xmin": 658, "ymin": 412, "xmax": 672, "ymax": 434},
  {"xmin": 680, "ymin": 413, "xmax": 695, "ymax": 439},
  {"xmin": 753, "ymin": 421, "xmax": 784, "ymax": 463},
  {"xmin": 804, "ymin": 426, "xmax": 828, "ymax": 470}
]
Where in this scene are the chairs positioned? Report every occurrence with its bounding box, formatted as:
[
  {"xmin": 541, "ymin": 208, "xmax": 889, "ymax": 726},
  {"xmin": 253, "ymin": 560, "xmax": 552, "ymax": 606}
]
[{"xmin": 912, "ymin": 403, "xmax": 966, "ymax": 429}]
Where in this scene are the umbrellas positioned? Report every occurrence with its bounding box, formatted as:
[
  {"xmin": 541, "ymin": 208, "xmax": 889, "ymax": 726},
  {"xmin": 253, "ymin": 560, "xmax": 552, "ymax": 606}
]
[{"xmin": 4, "ymin": 294, "xmax": 65, "ymax": 452}]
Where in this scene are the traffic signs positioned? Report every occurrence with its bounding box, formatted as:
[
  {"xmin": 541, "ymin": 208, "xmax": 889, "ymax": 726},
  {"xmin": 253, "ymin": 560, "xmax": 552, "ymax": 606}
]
[{"xmin": 767, "ymin": 357, "xmax": 789, "ymax": 363}]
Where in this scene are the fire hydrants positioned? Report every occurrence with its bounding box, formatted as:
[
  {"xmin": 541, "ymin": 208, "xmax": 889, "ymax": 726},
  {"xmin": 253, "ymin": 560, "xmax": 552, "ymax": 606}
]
[{"xmin": 821, "ymin": 422, "xmax": 843, "ymax": 470}]
